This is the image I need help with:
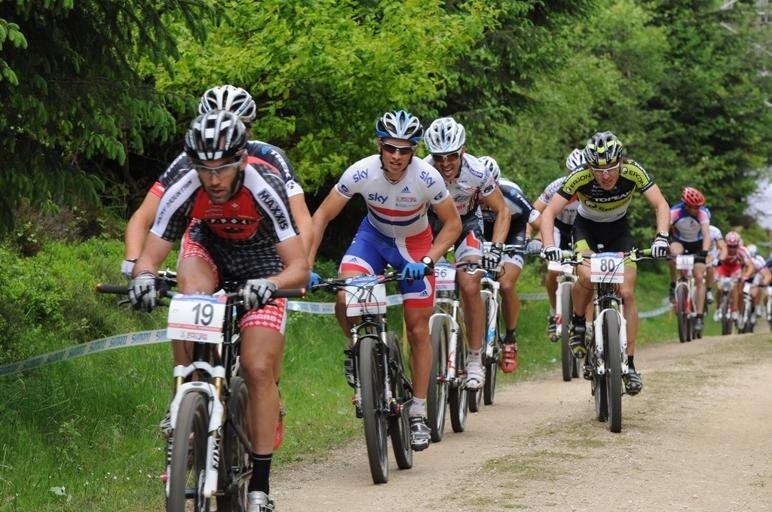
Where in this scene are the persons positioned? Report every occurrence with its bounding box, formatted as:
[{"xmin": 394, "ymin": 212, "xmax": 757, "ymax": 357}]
[
  {"xmin": 474, "ymin": 156, "xmax": 541, "ymax": 375},
  {"xmin": 421, "ymin": 116, "xmax": 512, "ymax": 391},
  {"xmin": 309, "ymin": 109, "xmax": 462, "ymax": 451},
  {"xmin": 539, "ymin": 130, "xmax": 670, "ymax": 396},
  {"xmin": 524, "ymin": 149, "xmax": 594, "ymax": 342},
  {"xmin": 702, "ymin": 208, "xmax": 772, "ymax": 324},
  {"xmin": 129, "ymin": 111, "xmax": 311, "ymax": 511},
  {"xmin": 670, "ymin": 187, "xmax": 710, "ymax": 330},
  {"xmin": 123, "ymin": 85, "xmax": 310, "ymax": 452}
]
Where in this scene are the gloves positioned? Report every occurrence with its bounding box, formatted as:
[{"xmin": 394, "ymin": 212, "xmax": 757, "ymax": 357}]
[
  {"xmin": 537, "ymin": 246, "xmax": 565, "ymax": 263},
  {"xmin": 307, "ymin": 270, "xmax": 323, "ymax": 291},
  {"xmin": 398, "ymin": 258, "xmax": 434, "ymax": 283},
  {"xmin": 647, "ymin": 234, "xmax": 671, "ymax": 260},
  {"xmin": 117, "ymin": 257, "xmax": 139, "ymax": 278},
  {"xmin": 478, "ymin": 242, "xmax": 506, "ymax": 271},
  {"xmin": 522, "ymin": 238, "xmax": 542, "ymax": 255},
  {"xmin": 237, "ymin": 275, "xmax": 278, "ymax": 313},
  {"xmin": 126, "ymin": 271, "xmax": 157, "ymax": 313}
]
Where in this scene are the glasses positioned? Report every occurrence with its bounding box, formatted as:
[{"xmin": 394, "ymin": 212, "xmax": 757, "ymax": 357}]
[
  {"xmin": 380, "ymin": 141, "xmax": 420, "ymax": 157},
  {"xmin": 587, "ymin": 161, "xmax": 622, "ymax": 177},
  {"xmin": 430, "ymin": 146, "xmax": 465, "ymax": 164},
  {"xmin": 187, "ymin": 149, "xmax": 248, "ymax": 179}
]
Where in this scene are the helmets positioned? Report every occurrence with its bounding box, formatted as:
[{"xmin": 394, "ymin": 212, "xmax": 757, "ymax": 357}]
[
  {"xmin": 180, "ymin": 109, "xmax": 249, "ymax": 163},
  {"xmin": 581, "ymin": 128, "xmax": 627, "ymax": 169},
  {"xmin": 196, "ymin": 84, "xmax": 259, "ymax": 123},
  {"xmin": 680, "ymin": 186, "xmax": 707, "ymax": 208},
  {"xmin": 421, "ymin": 113, "xmax": 469, "ymax": 156},
  {"xmin": 697, "ymin": 204, "xmax": 712, "ymax": 221},
  {"xmin": 374, "ymin": 108, "xmax": 424, "ymax": 144},
  {"xmin": 563, "ymin": 145, "xmax": 591, "ymax": 172},
  {"xmin": 725, "ymin": 231, "xmax": 741, "ymax": 246}
]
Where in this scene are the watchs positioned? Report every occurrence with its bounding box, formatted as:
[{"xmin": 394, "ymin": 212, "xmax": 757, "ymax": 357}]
[
  {"xmin": 493, "ymin": 242, "xmax": 503, "ymax": 250},
  {"xmin": 421, "ymin": 256, "xmax": 435, "ymax": 269},
  {"xmin": 656, "ymin": 231, "xmax": 669, "ymax": 240}
]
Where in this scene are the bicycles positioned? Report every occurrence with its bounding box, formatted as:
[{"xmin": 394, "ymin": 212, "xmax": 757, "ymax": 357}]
[{"xmin": 658, "ymin": 246, "xmax": 772, "ymax": 344}]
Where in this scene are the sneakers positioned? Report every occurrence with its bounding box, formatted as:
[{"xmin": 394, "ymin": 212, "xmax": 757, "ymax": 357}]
[
  {"xmin": 565, "ymin": 319, "xmax": 587, "ymax": 359},
  {"xmin": 461, "ymin": 355, "xmax": 488, "ymax": 391},
  {"xmin": 407, "ymin": 407, "xmax": 432, "ymax": 452},
  {"xmin": 622, "ymin": 359, "xmax": 643, "ymax": 397},
  {"xmin": 500, "ymin": 336, "xmax": 519, "ymax": 374},
  {"xmin": 341, "ymin": 343, "xmax": 356, "ymax": 387},
  {"xmin": 245, "ymin": 490, "xmax": 274, "ymax": 512},
  {"xmin": 694, "ymin": 306, "xmax": 772, "ymax": 331},
  {"xmin": 706, "ymin": 290, "xmax": 713, "ymax": 305},
  {"xmin": 545, "ymin": 311, "xmax": 562, "ymax": 343},
  {"xmin": 668, "ymin": 282, "xmax": 676, "ymax": 304},
  {"xmin": 158, "ymin": 409, "xmax": 173, "ymax": 436},
  {"xmin": 274, "ymin": 407, "xmax": 284, "ymax": 449}
]
